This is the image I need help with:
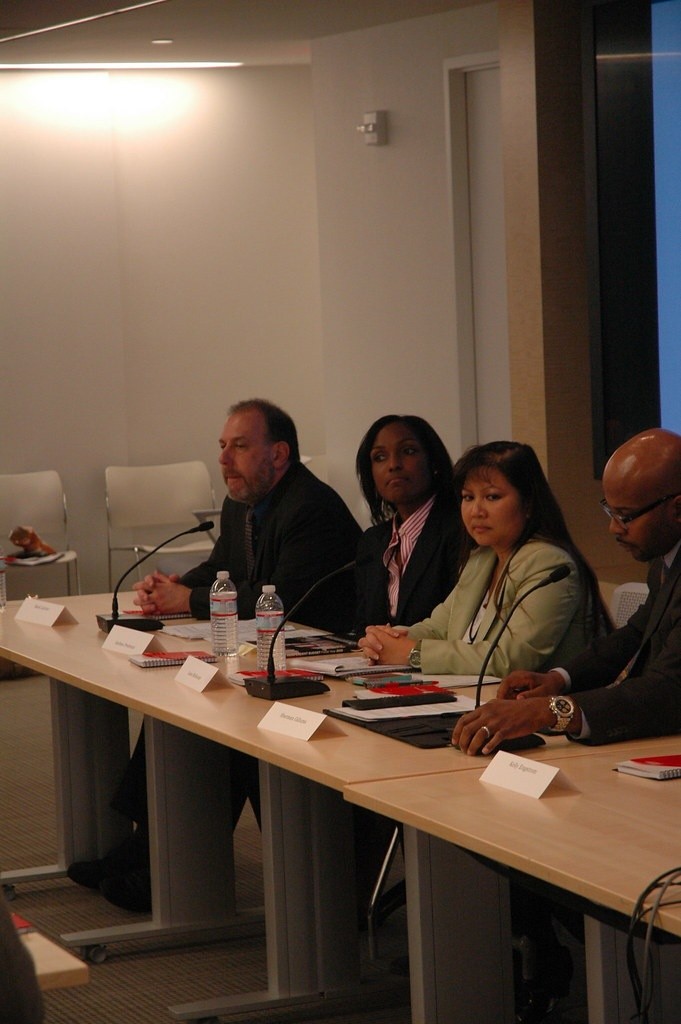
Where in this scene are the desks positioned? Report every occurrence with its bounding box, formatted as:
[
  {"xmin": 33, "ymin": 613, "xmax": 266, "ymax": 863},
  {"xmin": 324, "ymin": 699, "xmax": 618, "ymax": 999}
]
[{"xmin": 1, "ymin": 588, "xmax": 681, "ymax": 1024}]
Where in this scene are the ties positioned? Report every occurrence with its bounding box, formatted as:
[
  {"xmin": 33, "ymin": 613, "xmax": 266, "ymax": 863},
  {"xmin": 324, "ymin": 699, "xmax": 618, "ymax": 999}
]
[
  {"xmin": 243, "ymin": 505, "xmax": 255, "ymax": 584},
  {"xmin": 606, "ymin": 563, "xmax": 666, "ymax": 690}
]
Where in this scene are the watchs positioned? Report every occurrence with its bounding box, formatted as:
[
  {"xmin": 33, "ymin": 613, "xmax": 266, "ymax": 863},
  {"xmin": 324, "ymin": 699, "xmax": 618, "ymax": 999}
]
[
  {"xmin": 407, "ymin": 639, "xmax": 422, "ymax": 669},
  {"xmin": 548, "ymin": 694, "xmax": 574, "ymax": 732}
]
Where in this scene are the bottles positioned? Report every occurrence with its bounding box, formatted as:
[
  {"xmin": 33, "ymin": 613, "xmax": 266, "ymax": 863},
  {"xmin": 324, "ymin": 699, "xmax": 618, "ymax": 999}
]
[
  {"xmin": 255, "ymin": 585, "xmax": 287, "ymax": 674},
  {"xmin": 209, "ymin": 570, "xmax": 240, "ymax": 656},
  {"xmin": 0, "ymin": 551, "xmax": 7, "ymax": 613}
]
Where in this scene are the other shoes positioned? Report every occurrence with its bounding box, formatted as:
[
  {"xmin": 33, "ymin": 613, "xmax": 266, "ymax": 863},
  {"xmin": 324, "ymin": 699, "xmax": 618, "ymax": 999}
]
[
  {"xmin": 515, "ymin": 946, "xmax": 575, "ymax": 1024},
  {"xmin": 66, "ymin": 832, "xmax": 153, "ymax": 914}
]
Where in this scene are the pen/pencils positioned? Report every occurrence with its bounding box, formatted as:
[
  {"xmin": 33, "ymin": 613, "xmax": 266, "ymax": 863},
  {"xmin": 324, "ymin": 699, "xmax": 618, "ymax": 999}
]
[
  {"xmin": 512, "ymin": 688, "xmax": 528, "ymax": 692},
  {"xmin": 399, "ymin": 680, "xmax": 439, "ymax": 686}
]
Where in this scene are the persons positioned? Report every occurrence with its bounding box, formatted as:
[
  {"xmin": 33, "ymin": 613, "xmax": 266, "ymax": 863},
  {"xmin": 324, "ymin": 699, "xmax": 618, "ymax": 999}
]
[{"xmin": 68, "ymin": 399, "xmax": 681, "ymax": 999}]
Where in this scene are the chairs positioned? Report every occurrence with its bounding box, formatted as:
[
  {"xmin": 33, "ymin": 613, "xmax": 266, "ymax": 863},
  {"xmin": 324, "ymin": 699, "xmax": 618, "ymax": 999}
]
[
  {"xmin": 1, "ymin": 469, "xmax": 82, "ymax": 678},
  {"xmin": 610, "ymin": 582, "xmax": 652, "ymax": 676},
  {"xmin": 104, "ymin": 461, "xmax": 217, "ymax": 590}
]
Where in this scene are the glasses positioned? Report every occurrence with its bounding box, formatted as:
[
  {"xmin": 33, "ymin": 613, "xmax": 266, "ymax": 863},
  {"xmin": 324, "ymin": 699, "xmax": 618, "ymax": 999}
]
[{"xmin": 599, "ymin": 491, "xmax": 681, "ymax": 530}]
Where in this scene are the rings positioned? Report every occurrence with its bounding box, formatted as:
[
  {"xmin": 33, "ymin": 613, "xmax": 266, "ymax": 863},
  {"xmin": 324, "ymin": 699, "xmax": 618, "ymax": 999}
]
[{"xmin": 482, "ymin": 726, "xmax": 490, "ymax": 738}]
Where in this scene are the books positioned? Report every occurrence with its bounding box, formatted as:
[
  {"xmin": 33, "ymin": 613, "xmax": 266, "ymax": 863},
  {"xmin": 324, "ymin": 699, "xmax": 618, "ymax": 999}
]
[
  {"xmin": 611, "ymin": 754, "xmax": 681, "ymax": 781},
  {"xmin": 122, "ymin": 611, "xmax": 192, "ymax": 620},
  {"xmin": 128, "ymin": 651, "xmax": 218, "ymax": 668},
  {"xmin": 289, "ymin": 656, "xmax": 421, "ymax": 679},
  {"xmin": 246, "ymin": 632, "xmax": 363, "ymax": 657},
  {"xmin": 228, "ymin": 669, "xmax": 323, "ymax": 686},
  {"xmin": 352, "ymin": 684, "xmax": 456, "ymax": 700}
]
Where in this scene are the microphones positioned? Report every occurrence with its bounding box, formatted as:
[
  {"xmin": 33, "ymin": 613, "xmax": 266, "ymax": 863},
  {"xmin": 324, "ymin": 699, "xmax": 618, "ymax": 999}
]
[
  {"xmin": 243, "ymin": 552, "xmax": 373, "ymax": 701},
  {"xmin": 452, "ymin": 565, "xmax": 572, "ymax": 756},
  {"xmin": 95, "ymin": 521, "xmax": 214, "ymax": 634}
]
